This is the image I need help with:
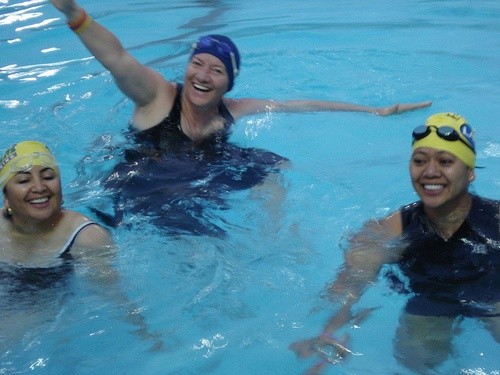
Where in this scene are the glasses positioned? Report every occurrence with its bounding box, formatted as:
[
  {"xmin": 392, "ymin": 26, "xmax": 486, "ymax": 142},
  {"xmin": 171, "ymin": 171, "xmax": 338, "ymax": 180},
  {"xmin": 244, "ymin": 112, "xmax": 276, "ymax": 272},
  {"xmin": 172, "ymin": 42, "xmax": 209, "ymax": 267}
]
[
  {"xmin": 0, "ymin": 152, "xmax": 56, "ymax": 185},
  {"xmin": 192, "ymin": 38, "xmax": 238, "ymax": 76},
  {"xmin": 413, "ymin": 125, "xmax": 475, "ymax": 152}
]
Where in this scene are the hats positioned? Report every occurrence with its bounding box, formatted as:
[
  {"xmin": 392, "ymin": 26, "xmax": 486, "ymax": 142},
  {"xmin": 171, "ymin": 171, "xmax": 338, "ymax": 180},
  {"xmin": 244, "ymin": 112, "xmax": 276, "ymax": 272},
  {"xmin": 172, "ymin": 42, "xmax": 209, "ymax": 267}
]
[
  {"xmin": 412, "ymin": 112, "xmax": 476, "ymax": 167},
  {"xmin": 190, "ymin": 34, "xmax": 240, "ymax": 92},
  {"xmin": 0, "ymin": 142, "xmax": 59, "ymax": 190}
]
